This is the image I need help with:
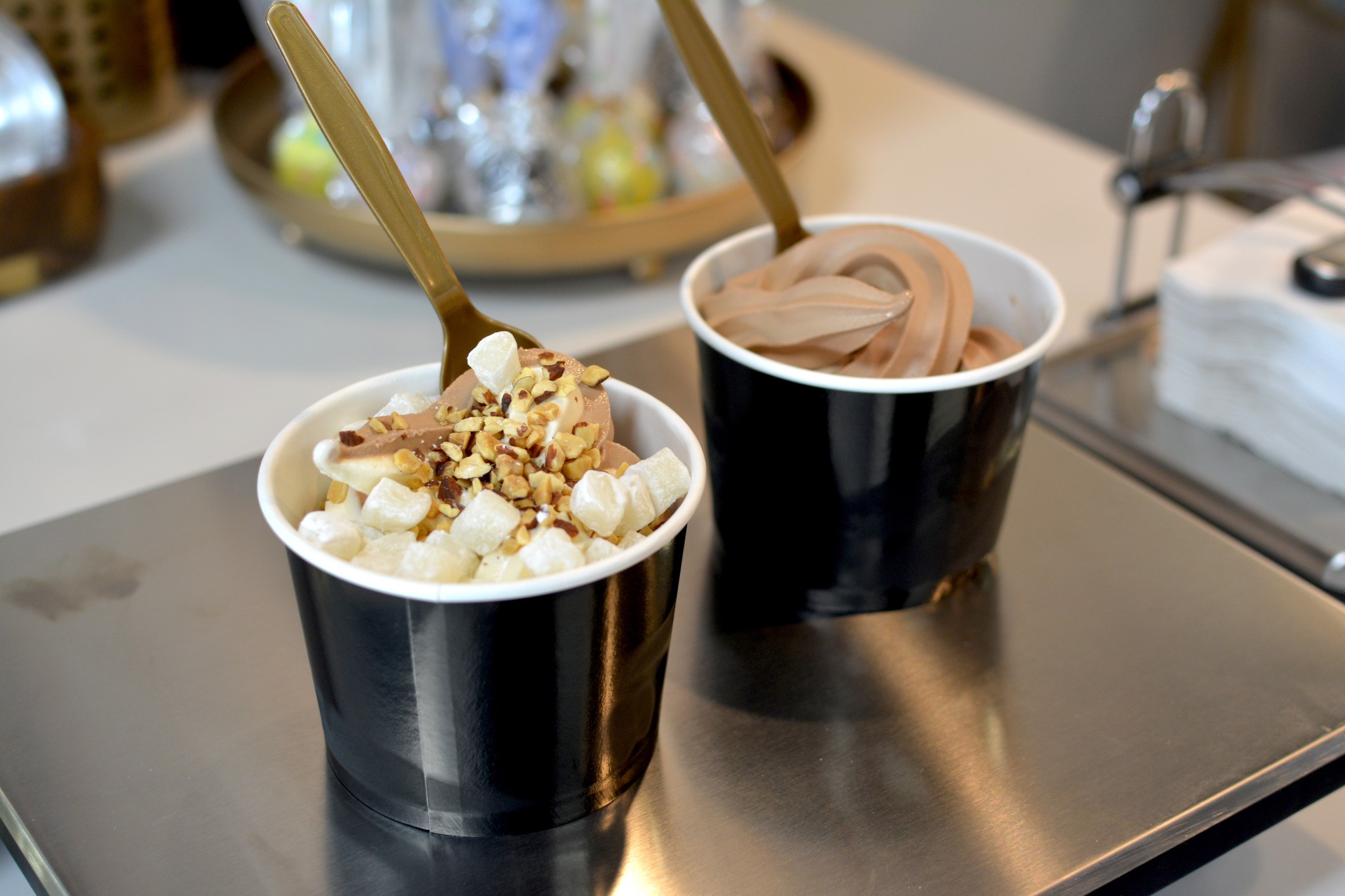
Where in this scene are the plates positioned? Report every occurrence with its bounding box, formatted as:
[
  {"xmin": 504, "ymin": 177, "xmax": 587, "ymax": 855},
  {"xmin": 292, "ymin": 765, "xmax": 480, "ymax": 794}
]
[{"xmin": 211, "ymin": 48, "xmax": 825, "ymax": 280}]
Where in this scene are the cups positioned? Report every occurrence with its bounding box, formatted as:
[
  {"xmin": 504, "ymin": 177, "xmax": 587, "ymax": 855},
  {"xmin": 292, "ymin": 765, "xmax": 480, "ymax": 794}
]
[
  {"xmin": 0, "ymin": 99, "xmax": 107, "ymax": 303},
  {"xmin": 679, "ymin": 215, "xmax": 1066, "ymax": 616},
  {"xmin": 255, "ymin": 363, "xmax": 707, "ymax": 839},
  {"xmin": 0, "ymin": 0, "xmax": 188, "ymax": 145}
]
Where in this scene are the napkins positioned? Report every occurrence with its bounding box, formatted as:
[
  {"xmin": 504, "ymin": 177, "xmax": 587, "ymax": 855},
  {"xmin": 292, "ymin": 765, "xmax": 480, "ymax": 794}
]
[{"xmin": 1152, "ymin": 190, "xmax": 1345, "ymax": 497}]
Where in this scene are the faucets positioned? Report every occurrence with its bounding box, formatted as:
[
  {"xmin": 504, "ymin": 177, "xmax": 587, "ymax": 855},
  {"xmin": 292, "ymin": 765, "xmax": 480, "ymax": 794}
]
[{"xmin": 1107, "ymin": 71, "xmax": 1205, "ymax": 313}]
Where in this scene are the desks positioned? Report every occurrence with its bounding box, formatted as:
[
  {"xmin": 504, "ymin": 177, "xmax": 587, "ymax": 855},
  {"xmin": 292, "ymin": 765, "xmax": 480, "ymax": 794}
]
[{"xmin": 0, "ymin": 7, "xmax": 1344, "ymax": 894}]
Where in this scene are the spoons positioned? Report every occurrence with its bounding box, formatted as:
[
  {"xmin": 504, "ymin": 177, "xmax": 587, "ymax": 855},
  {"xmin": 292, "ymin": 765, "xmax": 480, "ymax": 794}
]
[{"xmin": 264, "ymin": 0, "xmax": 545, "ymax": 395}]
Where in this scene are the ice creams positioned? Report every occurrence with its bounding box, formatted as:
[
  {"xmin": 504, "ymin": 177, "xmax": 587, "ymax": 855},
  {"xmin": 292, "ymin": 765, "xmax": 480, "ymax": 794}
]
[
  {"xmin": 297, "ymin": 330, "xmax": 691, "ymax": 584},
  {"xmin": 702, "ymin": 223, "xmax": 1021, "ymax": 378}
]
